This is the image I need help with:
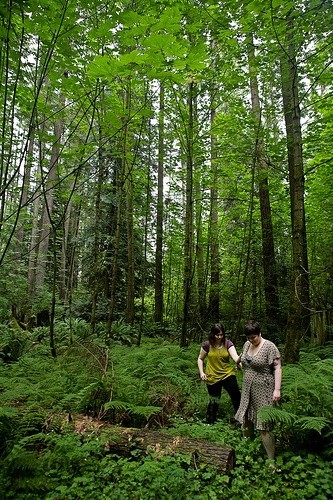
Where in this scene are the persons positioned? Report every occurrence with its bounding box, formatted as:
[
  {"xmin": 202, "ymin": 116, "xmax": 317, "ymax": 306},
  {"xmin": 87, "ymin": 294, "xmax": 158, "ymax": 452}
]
[
  {"xmin": 234, "ymin": 318, "xmax": 287, "ymax": 474},
  {"xmin": 198, "ymin": 322, "xmax": 248, "ymax": 428}
]
